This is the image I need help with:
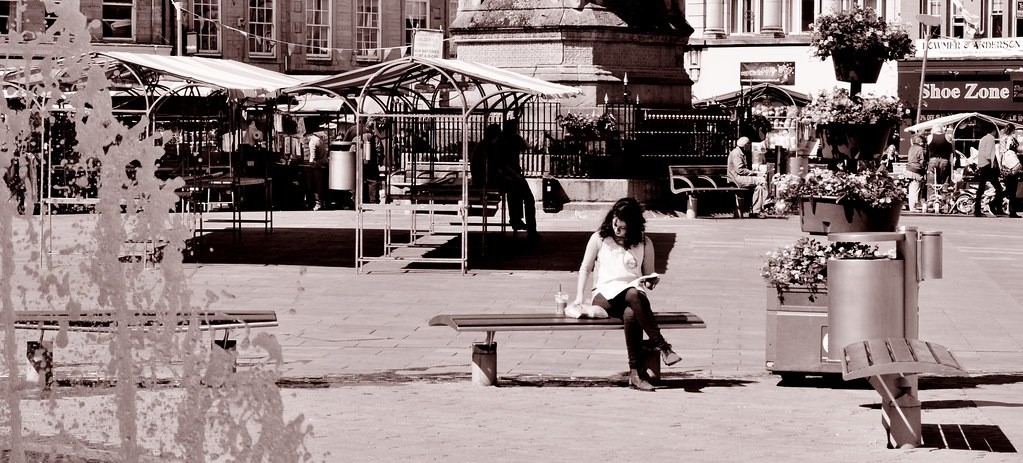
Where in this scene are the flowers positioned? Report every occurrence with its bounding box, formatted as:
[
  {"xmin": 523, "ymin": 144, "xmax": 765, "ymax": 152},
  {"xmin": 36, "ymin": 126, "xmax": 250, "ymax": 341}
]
[
  {"xmin": 770, "ymin": 165, "xmax": 907, "ymax": 208},
  {"xmin": 759, "ymin": 237, "xmax": 880, "ymax": 291},
  {"xmin": 809, "ymin": 8, "xmax": 916, "ymax": 61},
  {"xmin": 798, "ymin": 90, "xmax": 908, "ymax": 123}
]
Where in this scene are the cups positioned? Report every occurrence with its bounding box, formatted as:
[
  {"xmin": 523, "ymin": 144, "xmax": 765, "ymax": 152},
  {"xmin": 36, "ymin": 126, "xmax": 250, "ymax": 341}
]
[{"xmin": 555, "ymin": 291, "xmax": 569, "ymax": 315}]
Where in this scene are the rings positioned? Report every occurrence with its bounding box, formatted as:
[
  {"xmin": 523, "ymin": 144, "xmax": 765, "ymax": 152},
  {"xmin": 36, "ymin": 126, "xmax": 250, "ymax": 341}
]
[{"xmin": 653, "ymin": 285, "xmax": 655, "ymax": 287}]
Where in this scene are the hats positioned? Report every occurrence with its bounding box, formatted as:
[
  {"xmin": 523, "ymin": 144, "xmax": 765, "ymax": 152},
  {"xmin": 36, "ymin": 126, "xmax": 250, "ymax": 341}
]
[{"xmin": 737, "ymin": 137, "xmax": 749, "ymax": 147}]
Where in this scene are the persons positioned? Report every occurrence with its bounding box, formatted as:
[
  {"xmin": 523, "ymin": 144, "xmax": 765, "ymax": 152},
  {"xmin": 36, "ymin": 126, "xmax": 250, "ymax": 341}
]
[
  {"xmin": 470, "ymin": 118, "xmax": 543, "ymax": 239},
  {"xmin": 571, "ymin": 197, "xmax": 683, "ymax": 392},
  {"xmin": 727, "ymin": 136, "xmax": 769, "ymax": 219},
  {"xmin": 907, "ymin": 135, "xmax": 930, "ymax": 213},
  {"xmin": 828, "ymin": 139, "xmax": 899, "ymax": 174},
  {"xmin": 303, "ymin": 130, "xmax": 385, "ymax": 212},
  {"xmin": 974, "ymin": 123, "xmax": 1023, "ymax": 218}
]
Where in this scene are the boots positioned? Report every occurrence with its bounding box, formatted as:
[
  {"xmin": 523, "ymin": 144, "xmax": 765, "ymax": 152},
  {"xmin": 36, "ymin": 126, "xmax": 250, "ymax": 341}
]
[
  {"xmin": 629, "ymin": 368, "xmax": 655, "ymax": 391},
  {"xmin": 659, "ymin": 341, "xmax": 681, "ymax": 366}
]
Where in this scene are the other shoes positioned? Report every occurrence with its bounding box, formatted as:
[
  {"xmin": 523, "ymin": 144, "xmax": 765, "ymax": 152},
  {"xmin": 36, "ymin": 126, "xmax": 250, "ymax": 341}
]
[
  {"xmin": 988, "ymin": 201, "xmax": 999, "ymax": 215},
  {"xmin": 1009, "ymin": 213, "xmax": 1022, "ymax": 218},
  {"xmin": 911, "ymin": 209, "xmax": 922, "ymax": 213},
  {"xmin": 527, "ymin": 232, "xmax": 543, "ymax": 241},
  {"xmin": 974, "ymin": 213, "xmax": 987, "ymax": 217},
  {"xmin": 754, "ymin": 213, "xmax": 766, "ymax": 218},
  {"xmin": 996, "ymin": 208, "xmax": 1008, "ymax": 216},
  {"xmin": 510, "ymin": 219, "xmax": 527, "ymax": 231}
]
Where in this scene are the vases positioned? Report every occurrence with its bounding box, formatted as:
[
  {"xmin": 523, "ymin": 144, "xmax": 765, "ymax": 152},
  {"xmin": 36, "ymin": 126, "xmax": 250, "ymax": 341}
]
[
  {"xmin": 831, "ymin": 50, "xmax": 883, "ymax": 84},
  {"xmin": 796, "ymin": 193, "xmax": 903, "ymax": 232},
  {"xmin": 817, "ymin": 123, "xmax": 891, "ymax": 165}
]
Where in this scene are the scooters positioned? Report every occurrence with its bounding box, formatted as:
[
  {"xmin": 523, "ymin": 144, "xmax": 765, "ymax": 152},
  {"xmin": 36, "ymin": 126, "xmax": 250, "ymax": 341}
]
[{"xmin": 943, "ymin": 170, "xmax": 1010, "ymax": 216}]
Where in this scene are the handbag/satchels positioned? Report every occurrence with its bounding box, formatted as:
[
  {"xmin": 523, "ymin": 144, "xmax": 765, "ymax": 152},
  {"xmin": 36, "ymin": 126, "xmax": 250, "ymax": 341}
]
[{"xmin": 1002, "ymin": 150, "xmax": 1021, "ymax": 175}]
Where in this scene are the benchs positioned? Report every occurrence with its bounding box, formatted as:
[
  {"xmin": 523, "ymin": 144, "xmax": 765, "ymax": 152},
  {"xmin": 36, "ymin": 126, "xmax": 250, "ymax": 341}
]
[
  {"xmin": 427, "ymin": 311, "xmax": 707, "ymax": 384},
  {"xmin": 670, "ymin": 164, "xmax": 754, "ymax": 218},
  {"xmin": 0, "ymin": 310, "xmax": 278, "ymax": 392},
  {"xmin": 839, "ymin": 338, "xmax": 968, "ymax": 448}
]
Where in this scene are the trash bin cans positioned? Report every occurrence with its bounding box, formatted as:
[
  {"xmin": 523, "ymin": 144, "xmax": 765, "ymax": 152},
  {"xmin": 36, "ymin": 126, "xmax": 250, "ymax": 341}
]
[
  {"xmin": 825, "ymin": 232, "xmax": 906, "ymax": 364},
  {"xmin": 328, "ymin": 140, "xmax": 354, "ymax": 191}
]
